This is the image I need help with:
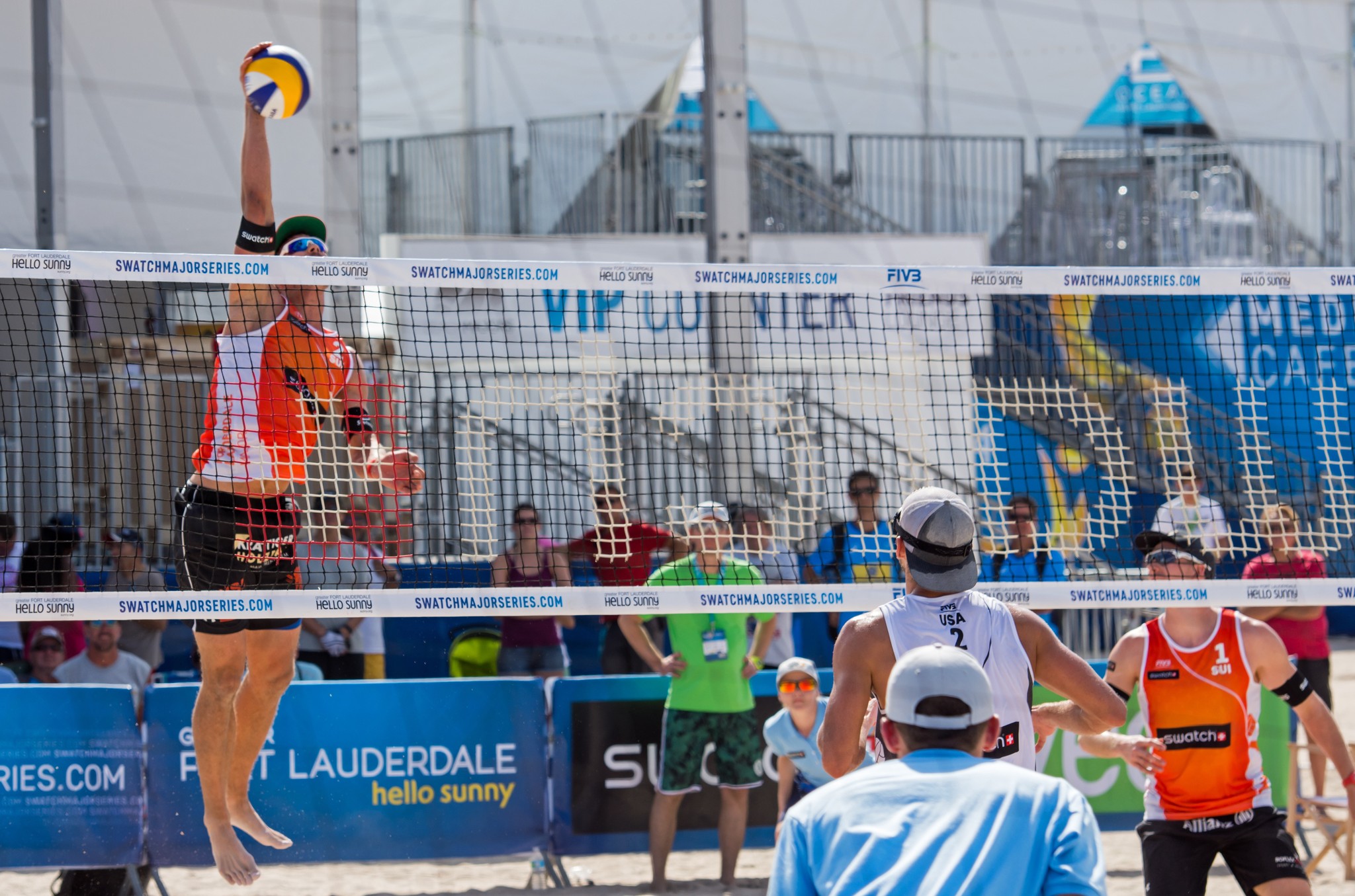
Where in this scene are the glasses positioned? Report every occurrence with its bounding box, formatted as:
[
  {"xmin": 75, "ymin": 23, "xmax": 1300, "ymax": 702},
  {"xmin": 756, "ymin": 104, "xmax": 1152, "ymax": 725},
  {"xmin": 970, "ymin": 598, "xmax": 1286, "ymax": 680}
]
[
  {"xmin": 279, "ymin": 236, "xmax": 330, "ymax": 256},
  {"xmin": 89, "ymin": 620, "xmax": 117, "ymax": 626},
  {"xmin": 514, "ymin": 517, "xmax": 539, "ymax": 525},
  {"xmin": 1008, "ymin": 513, "xmax": 1034, "ymax": 521},
  {"xmin": 32, "ymin": 644, "xmax": 65, "ymax": 652},
  {"xmin": 1264, "ymin": 523, "xmax": 1294, "ymax": 534},
  {"xmin": 694, "ymin": 522, "xmax": 729, "ymax": 535},
  {"xmin": 1144, "ymin": 548, "xmax": 1212, "ymax": 572},
  {"xmin": 596, "ymin": 498, "xmax": 620, "ymax": 507},
  {"xmin": 1181, "ymin": 471, "xmax": 1198, "ymax": 480},
  {"xmin": 850, "ymin": 486, "xmax": 877, "ymax": 497},
  {"xmin": 108, "ymin": 543, "xmax": 125, "ymax": 550},
  {"xmin": 778, "ymin": 678, "xmax": 817, "ymax": 694}
]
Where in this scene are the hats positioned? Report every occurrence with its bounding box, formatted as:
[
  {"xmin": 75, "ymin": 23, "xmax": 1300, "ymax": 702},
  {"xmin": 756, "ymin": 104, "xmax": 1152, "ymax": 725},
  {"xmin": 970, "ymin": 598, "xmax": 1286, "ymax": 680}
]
[
  {"xmin": 885, "ymin": 642, "xmax": 995, "ymax": 729},
  {"xmin": 896, "ymin": 485, "xmax": 978, "ymax": 593},
  {"xmin": 48, "ymin": 511, "xmax": 84, "ymax": 536},
  {"xmin": 1134, "ymin": 528, "xmax": 1216, "ymax": 580},
  {"xmin": 274, "ymin": 215, "xmax": 327, "ymax": 256},
  {"xmin": 100, "ymin": 528, "xmax": 144, "ymax": 549},
  {"xmin": 775, "ymin": 657, "xmax": 819, "ymax": 690},
  {"xmin": 31, "ymin": 626, "xmax": 64, "ymax": 647},
  {"xmin": 688, "ymin": 500, "xmax": 730, "ymax": 527}
]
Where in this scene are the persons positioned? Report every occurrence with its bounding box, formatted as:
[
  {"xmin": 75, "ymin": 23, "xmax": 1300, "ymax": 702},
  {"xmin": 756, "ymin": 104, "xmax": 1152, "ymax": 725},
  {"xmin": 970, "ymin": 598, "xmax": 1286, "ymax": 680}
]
[
  {"xmin": 0, "ymin": 488, "xmax": 401, "ymax": 684},
  {"xmin": 618, "ymin": 499, "xmax": 802, "ymax": 890},
  {"xmin": 174, "ymin": 41, "xmax": 427, "ymax": 887},
  {"xmin": 803, "ymin": 470, "xmax": 906, "ymax": 643},
  {"xmin": 984, "ymin": 497, "xmax": 1072, "ymax": 637},
  {"xmin": 551, "ymin": 486, "xmax": 689, "ymax": 674},
  {"xmin": 1239, "ymin": 501, "xmax": 1333, "ymax": 817},
  {"xmin": 766, "ymin": 642, "xmax": 1107, "ymax": 896},
  {"xmin": 1150, "ymin": 464, "xmax": 1232, "ymax": 575},
  {"xmin": 763, "ymin": 657, "xmax": 876, "ymax": 844},
  {"xmin": 491, "ymin": 504, "xmax": 576, "ymax": 676},
  {"xmin": 816, "ymin": 487, "xmax": 1127, "ymax": 778},
  {"xmin": 1077, "ymin": 530, "xmax": 1355, "ymax": 896}
]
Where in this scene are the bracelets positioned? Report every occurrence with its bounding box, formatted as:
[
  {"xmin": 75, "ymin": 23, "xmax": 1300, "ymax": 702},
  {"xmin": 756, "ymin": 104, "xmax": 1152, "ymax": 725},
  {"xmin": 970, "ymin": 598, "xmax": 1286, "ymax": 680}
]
[
  {"xmin": 366, "ymin": 457, "xmax": 379, "ymax": 480},
  {"xmin": 749, "ymin": 655, "xmax": 763, "ymax": 669},
  {"xmin": 1342, "ymin": 770, "xmax": 1355, "ymax": 787}
]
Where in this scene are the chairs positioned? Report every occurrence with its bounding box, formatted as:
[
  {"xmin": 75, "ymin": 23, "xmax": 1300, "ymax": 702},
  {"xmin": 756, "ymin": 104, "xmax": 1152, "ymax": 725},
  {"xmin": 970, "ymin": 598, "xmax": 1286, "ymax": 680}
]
[{"xmin": 1286, "ymin": 739, "xmax": 1355, "ymax": 881}]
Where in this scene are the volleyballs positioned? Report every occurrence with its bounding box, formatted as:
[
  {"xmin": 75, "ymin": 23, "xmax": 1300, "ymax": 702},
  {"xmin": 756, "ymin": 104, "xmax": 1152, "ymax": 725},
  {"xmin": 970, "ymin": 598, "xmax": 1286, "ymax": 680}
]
[{"xmin": 244, "ymin": 45, "xmax": 313, "ymax": 119}]
